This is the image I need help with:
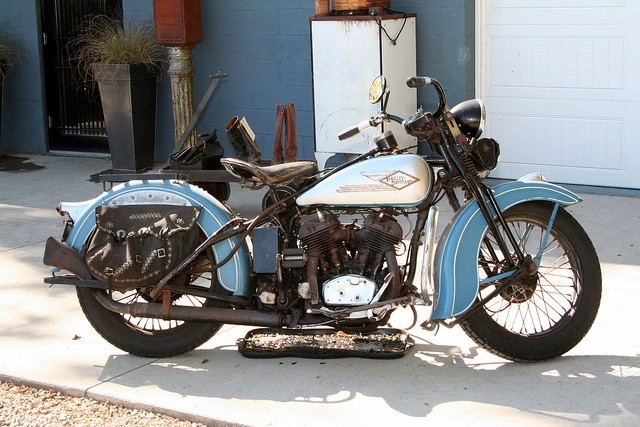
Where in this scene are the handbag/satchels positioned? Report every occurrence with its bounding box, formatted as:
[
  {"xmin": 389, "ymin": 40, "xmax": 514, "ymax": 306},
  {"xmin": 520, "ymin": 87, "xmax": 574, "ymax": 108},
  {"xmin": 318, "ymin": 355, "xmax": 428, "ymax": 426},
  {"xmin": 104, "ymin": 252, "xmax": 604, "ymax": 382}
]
[{"xmin": 84, "ymin": 205, "xmax": 202, "ymax": 289}]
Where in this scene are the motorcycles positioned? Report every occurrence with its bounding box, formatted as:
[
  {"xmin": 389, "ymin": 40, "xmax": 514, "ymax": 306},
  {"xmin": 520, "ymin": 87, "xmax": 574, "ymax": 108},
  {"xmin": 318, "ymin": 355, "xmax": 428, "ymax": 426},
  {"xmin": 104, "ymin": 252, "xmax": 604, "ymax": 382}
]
[{"xmin": 43, "ymin": 75, "xmax": 604, "ymax": 364}]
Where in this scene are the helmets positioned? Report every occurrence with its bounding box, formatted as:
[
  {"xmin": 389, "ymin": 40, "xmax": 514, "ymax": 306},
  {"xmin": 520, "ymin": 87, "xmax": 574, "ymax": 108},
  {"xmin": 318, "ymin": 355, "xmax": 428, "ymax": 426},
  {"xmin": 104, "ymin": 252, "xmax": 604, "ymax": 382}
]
[{"xmin": 159, "ymin": 129, "xmax": 230, "ymax": 205}]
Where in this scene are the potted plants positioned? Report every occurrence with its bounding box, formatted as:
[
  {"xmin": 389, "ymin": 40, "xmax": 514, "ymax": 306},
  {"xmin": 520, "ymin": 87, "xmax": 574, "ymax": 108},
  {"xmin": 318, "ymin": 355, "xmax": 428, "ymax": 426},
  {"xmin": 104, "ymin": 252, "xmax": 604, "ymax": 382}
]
[{"xmin": 64, "ymin": 12, "xmax": 170, "ymax": 173}]
